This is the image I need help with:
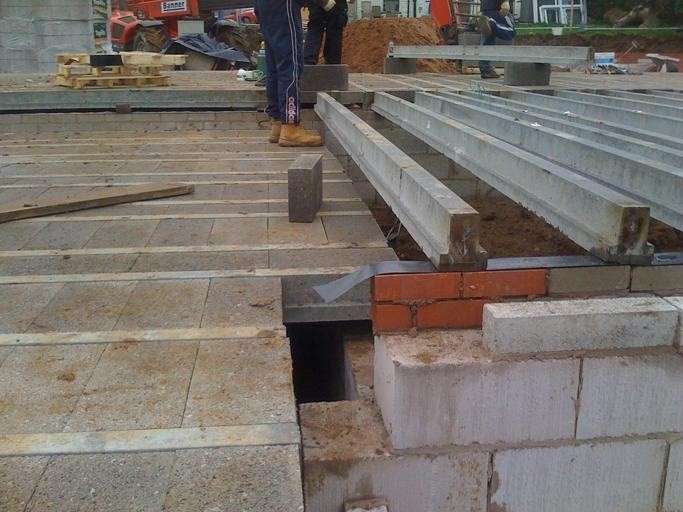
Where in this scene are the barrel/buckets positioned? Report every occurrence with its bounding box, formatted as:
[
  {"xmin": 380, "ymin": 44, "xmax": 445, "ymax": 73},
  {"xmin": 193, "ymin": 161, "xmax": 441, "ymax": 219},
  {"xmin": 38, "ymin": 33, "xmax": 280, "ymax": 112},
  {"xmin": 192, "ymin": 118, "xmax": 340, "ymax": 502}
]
[
  {"xmin": 552, "ymin": 26, "xmax": 563, "ymax": 36},
  {"xmin": 594, "ymin": 52, "xmax": 615, "ymax": 67}
]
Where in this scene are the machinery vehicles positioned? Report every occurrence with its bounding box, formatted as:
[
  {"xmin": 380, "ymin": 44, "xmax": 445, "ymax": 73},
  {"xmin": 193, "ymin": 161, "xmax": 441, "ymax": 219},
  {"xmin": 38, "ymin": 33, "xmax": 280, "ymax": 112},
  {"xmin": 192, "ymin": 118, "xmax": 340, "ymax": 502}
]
[{"xmin": 107, "ymin": 0, "xmax": 262, "ymax": 71}]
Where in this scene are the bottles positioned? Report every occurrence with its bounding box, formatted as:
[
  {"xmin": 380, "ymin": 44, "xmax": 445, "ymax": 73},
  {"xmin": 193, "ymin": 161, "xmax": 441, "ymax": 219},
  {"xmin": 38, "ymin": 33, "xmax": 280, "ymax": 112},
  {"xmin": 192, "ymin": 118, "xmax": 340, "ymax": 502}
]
[{"xmin": 257, "ymin": 48, "xmax": 267, "ymax": 75}]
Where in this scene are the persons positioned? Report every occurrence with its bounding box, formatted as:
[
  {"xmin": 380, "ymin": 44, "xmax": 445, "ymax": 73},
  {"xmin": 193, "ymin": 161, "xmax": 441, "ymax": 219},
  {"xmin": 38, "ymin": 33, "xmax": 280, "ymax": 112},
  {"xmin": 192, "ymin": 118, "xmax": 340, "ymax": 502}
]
[
  {"xmin": 301, "ymin": 0, "xmax": 349, "ymax": 65},
  {"xmin": 251, "ymin": 0, "xmax": 325, "ymax": 148},
  {"xmin": 476, "ymin": 0, "xmax": 517, "ymax": 79}
]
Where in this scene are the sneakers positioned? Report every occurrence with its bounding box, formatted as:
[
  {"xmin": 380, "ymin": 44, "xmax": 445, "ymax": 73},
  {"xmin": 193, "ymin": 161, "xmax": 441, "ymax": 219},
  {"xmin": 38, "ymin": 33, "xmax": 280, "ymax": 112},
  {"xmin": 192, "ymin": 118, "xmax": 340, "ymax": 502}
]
[
  {"xmin": 480, "ymin": 16, "xmax": 492, "ymax": 37},
  {"xmin": 269, "ymin": 120, "xmax": 322, "ymax": 147},
  {"xmin": 481, "ymin": 66, "xmax": 501, "ymax": 79}
]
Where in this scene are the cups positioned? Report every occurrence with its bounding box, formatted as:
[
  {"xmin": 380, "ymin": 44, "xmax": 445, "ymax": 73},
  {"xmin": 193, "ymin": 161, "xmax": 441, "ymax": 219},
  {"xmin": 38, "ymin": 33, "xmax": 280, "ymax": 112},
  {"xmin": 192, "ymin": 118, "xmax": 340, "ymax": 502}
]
[
  {"xmin": 253, "ymin": 70, "xmax": 263, "ymax": 80},
  {"xmin": 246, "ymin": 71, "xmax": 252, "ymax": 79}
]
